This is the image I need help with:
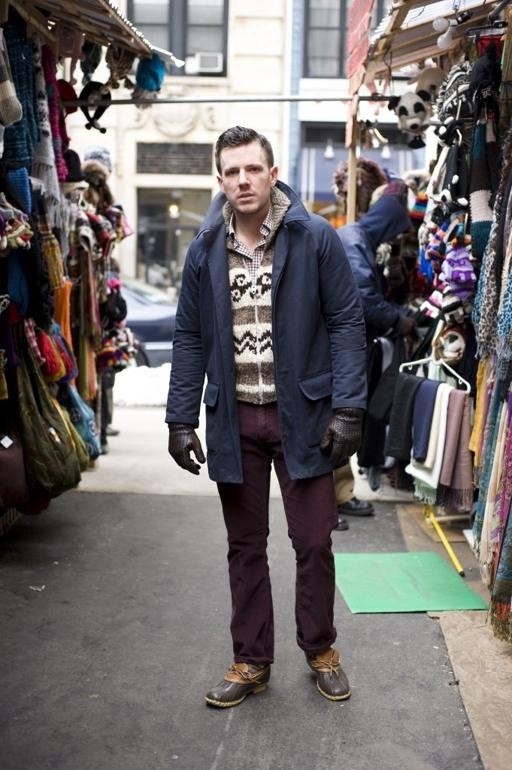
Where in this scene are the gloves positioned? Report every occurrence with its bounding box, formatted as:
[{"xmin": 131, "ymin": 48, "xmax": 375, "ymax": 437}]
[
  {"xmin": 418, "ymin": 282, "xmax": 450, "ymax": 319},
  {"xmin": 321, "ymin": 409, "xmax": 362, "ymax": 465},
  {"xmin": 445, "ymin": 248, "xmax": 477, "ymax": 303},
  {"xmin": 168, "ymin": 424, "xmax": 206, "ymax": 476},
  {"xmin": 442, "ymin": 286, "xmax": 473, "ymax": 327}
]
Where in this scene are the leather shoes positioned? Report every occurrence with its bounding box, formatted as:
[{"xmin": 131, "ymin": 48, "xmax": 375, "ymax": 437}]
[
  {"xmin": 337, "ymin": 498, "xmax": 374, "ymax": 515},
  {"xmin": 335, "ymin": 516, "xmax": 350, "ymax": 530}
]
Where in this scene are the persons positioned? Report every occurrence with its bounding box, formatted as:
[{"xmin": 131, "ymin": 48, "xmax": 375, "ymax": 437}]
[
  {"xmin": 331, "ymin": 195, "xmax": 422, "ymax": 530},
  {"xmin": 164, "ymin": 125, "xmax": 370, "ymax": 708}
]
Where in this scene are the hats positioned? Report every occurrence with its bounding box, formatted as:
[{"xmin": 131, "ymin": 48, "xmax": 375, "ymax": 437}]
[
  {"xmin": 434, "ymin": 61, "xmax": 471, "ymax": 147},
  {"xmin": 95, "ymin": 338, "xmax": 129, "ymax": 375},
  {"xmin": 469, "ymin": 49, "xmax": 502, "ymax": 125},
  {"xmin": 75, "ymin": 203, "xmax": 134, "ymax": 303},
  {"xmin": 58, "ymin": 150, "xmax": 82, "ymax": 182},
  {"xmin": 83, "ymin": 148, "xmax": 112, "ymax": 180},
  {"xmin": 53, "ymin": 21, "xmax": 167, "ymax": 132},
  {"xmin": 442, "ymin": 143, "xmax": 472, "ymax": 206}
]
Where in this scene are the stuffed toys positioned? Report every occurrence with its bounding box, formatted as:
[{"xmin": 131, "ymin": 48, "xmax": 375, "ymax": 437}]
[
  {"xmin": 408, "ymin": 67, "xmax": 447, "ymax": 101},
  {"xmin": 386, "ymin": 90, "xmax": 431, "ymax": 135}
]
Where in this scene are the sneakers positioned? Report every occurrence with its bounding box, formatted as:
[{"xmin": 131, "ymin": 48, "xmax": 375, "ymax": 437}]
[
  {"xmin": 306, "ymin": 648, "xmax": 351, "ymax": 701},
  {"xmin": 204, "ymin": 663, "xmax": 271, "ymax": 707},
  {"xmin": 106, "ymin": 425, "xmax": 119, "ymax": 435}
]
